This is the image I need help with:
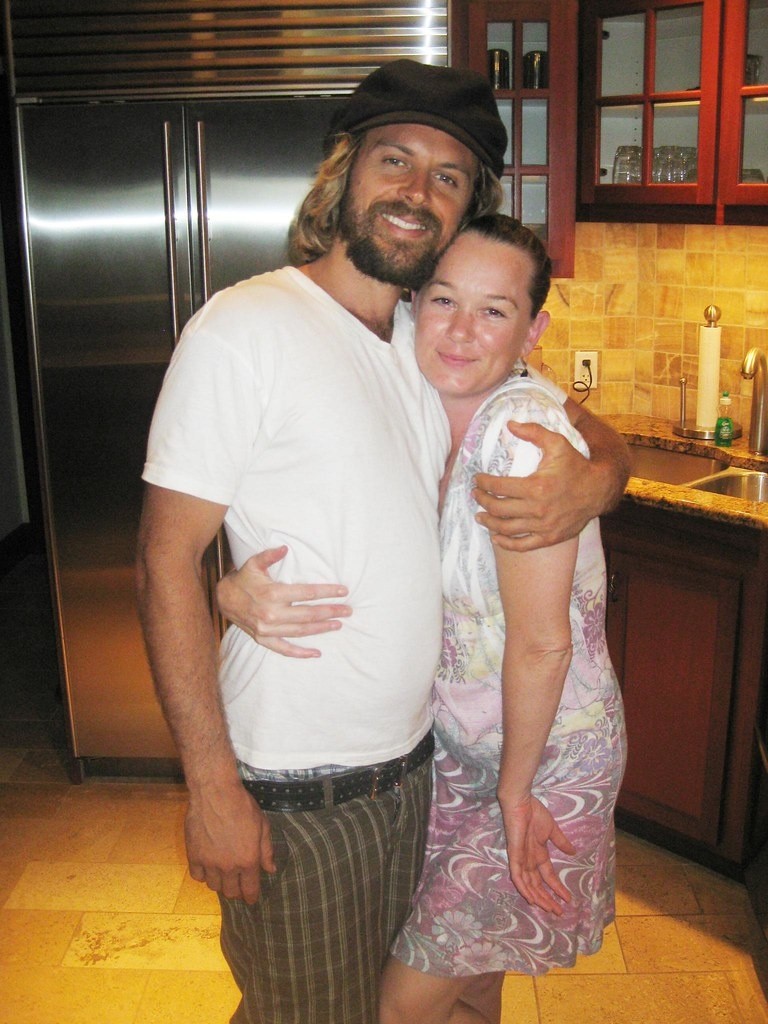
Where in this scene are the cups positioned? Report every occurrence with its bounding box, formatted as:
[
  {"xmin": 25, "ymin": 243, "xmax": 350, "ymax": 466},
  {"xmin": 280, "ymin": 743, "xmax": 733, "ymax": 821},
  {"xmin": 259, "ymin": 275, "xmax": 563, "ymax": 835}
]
[
  {"xmin": 488, "ymin": 49, "xmax": 511, "ymax": 89},
  {"xmin": 613, "ymin": 145, "xmax": 699, "ymax": 184},
  {"xmin": 743, "ymin": 53, "xmax": 763, "ymax": 84},
  {"xmin": 524, "ymin": 51, "xmax": 549, "ymax": 88}
]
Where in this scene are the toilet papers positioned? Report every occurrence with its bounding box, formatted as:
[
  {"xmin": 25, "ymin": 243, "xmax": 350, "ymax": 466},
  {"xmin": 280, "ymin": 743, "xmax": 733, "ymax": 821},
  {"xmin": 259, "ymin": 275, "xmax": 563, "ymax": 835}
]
[{"xmin": 696, "ymin": 325, "xmax": 722, "ymax": 428}]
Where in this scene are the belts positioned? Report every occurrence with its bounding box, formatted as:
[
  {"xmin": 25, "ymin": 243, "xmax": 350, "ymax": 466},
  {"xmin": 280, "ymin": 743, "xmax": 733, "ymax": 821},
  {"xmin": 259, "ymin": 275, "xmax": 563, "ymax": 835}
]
[{"xmin": 239, "ymin": 723, "xmax": 437, "ymax": 815}]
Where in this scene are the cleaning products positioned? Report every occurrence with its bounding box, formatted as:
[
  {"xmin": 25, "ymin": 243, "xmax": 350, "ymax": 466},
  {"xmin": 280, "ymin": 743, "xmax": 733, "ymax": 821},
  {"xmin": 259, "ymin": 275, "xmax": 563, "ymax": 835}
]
[{"xmin": 715, "ymin": 391, "xmax": 733, "ymax": 447}]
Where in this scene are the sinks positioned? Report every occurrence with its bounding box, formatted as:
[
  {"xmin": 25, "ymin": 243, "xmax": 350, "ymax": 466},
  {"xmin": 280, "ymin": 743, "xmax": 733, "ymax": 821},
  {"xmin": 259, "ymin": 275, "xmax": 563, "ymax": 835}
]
[
  {"xmin": 684, "ymin": 457, "xmax": 768, "ymax": 503},
  {"xmin": 615, "ymin": 432, "xmax": 732, "ymax": 486}
]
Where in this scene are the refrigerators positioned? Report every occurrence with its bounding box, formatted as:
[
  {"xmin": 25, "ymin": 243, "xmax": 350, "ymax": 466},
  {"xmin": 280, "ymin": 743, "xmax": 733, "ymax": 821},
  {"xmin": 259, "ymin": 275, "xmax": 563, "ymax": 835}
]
[{"xmin": 17, "ymin": 98, "xmax": 359, "ymax": 787}]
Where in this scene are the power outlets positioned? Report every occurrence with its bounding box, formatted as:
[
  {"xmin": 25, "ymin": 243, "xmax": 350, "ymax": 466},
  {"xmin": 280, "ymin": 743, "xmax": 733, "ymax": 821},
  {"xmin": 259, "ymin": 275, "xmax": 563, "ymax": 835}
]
[{"xmin": 575, "ymin": 351, "xmax": 598, "ymax": 389}]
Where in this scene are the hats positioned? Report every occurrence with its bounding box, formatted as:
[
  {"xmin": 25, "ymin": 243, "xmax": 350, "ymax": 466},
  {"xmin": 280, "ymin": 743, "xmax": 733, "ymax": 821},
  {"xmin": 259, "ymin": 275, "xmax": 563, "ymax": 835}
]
[{"xmin": 321, "ymin": 58, "xmax": 508, "ymax": 182}]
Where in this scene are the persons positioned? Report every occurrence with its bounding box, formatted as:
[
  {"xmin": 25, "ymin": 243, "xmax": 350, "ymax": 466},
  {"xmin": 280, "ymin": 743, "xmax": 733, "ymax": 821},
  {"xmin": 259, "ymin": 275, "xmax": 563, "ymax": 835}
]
[
  {"xmin": 131, "ymin": 59, "xmax": 629, "ymax": 1024},
  {"xmin": 216, "ymin": 213, "xmax": 628, "ymax": 1024}
]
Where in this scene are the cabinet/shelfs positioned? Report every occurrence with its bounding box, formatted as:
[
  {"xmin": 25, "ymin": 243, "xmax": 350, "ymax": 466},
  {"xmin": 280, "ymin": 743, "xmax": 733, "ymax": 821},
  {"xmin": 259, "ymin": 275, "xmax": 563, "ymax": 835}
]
[
  {"xmin": 603, "ymin": 546, "xmax": 768, "ymax": 884},
  {"xmin": 579, "ymin": 0, "xmax": 767, "ymax": 225},
  {"xmin": 467, "ymin": 1, "xmax": 577, "ymax": 279}
]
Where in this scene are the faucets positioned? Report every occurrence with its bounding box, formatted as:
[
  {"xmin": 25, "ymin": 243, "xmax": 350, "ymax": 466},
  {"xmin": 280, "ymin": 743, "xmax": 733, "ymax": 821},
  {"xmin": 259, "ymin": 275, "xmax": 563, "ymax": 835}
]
[{"xmin": 740, "ymin": 346, "xmax": 768, "ymax": 456}]
